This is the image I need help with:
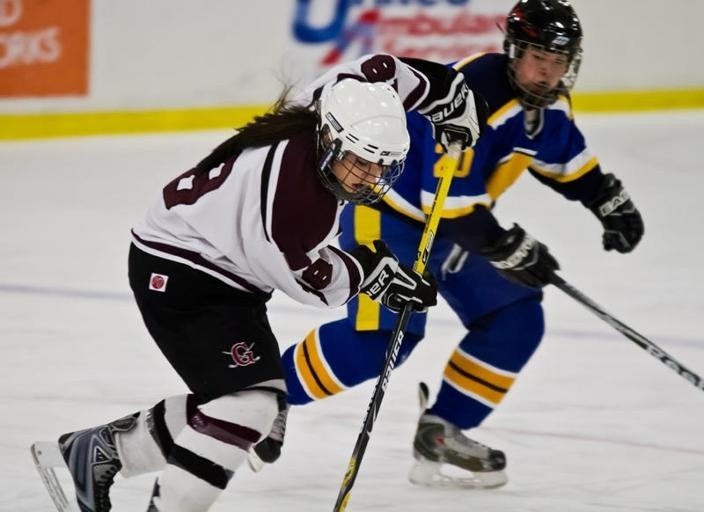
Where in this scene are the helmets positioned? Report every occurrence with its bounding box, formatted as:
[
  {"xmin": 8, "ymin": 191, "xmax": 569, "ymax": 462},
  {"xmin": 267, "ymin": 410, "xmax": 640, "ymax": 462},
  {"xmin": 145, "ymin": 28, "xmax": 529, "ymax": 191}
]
[
  {"xmin": 317, "ymin": 79, "xmax": 409, "ymax": 207},
  {"xmin": 504, "ymin": 0, "xmax": 584, "ymax": 110}
]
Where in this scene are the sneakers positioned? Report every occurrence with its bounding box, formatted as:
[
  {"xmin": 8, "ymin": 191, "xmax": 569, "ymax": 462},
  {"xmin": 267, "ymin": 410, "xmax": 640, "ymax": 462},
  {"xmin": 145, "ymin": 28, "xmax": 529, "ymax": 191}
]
[{"xmin": 253, "ymin": 391, "xmax": 290, "ymax": 463}]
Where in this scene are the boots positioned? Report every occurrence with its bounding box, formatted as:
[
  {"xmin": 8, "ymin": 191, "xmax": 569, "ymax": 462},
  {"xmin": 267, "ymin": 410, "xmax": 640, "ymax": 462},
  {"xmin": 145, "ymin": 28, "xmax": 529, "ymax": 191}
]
[
  {"xmin": 413, "ymin": 381, "xmax": 506, "ymax": 472},
  {"xmin": 58, "ymin": 412, "xmax": 140, "ymax": 512}
]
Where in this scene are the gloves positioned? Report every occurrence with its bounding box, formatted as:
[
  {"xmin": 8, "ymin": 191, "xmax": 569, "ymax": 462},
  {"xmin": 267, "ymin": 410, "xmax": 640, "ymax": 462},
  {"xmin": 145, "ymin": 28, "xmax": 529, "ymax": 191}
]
[
  {"xmin": 487, "ymin": 223, "xmax": 560, "ymax": 289},
  {"xmin": 422, "ymin": 73, "xmax": 478, "ymax": 151},
  {"xmin": 582, "ymin": 172, "xmax": 645, "ymax": 253},
  {"xmin": 357, "ymin": 240, "xmax": 436, "ymax": 313}
]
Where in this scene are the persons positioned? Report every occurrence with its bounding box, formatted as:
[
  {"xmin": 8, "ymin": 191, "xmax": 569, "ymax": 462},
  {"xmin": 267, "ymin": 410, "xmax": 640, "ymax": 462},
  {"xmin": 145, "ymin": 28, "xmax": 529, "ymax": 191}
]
[
  {"xmin": 56, "ymin": 50, "xmax": 482, "ymax": 512},
  {"xmin": 251, "ymin": 1, "xmax": 646, "ymax": 473}
]
